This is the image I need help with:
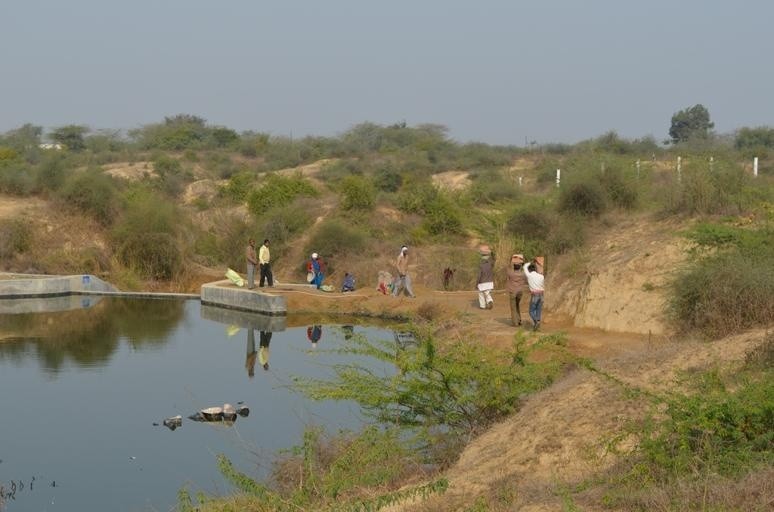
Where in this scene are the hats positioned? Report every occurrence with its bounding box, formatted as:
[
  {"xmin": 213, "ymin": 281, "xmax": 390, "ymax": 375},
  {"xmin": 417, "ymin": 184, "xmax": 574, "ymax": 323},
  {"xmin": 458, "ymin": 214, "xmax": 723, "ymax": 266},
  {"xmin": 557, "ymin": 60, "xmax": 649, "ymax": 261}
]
[{"xmin": 312, "ymin": 252, "xmax": 318, "ymax": 259}]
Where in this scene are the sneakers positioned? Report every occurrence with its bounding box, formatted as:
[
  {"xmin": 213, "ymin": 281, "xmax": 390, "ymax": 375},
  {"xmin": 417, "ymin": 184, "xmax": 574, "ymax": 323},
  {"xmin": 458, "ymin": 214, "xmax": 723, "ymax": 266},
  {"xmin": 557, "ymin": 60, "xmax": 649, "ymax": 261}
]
[{"xmin": 480, "ymin": 301, "xmax": 540, "ymax": 331}]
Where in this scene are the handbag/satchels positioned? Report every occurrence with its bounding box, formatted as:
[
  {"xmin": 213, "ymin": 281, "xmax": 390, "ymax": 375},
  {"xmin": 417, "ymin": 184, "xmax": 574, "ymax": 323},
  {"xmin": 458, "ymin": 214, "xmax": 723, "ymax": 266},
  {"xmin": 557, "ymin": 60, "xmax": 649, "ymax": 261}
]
[{"xmin": 306, "ymin": 272, "xmax": 315, "ymax": 282}]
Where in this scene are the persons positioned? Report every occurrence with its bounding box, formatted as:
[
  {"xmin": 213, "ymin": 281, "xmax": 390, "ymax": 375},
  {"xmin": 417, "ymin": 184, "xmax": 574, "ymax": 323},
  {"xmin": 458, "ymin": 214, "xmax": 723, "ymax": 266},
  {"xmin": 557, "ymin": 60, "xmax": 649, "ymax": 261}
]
[
  {"xmin": 392, "ymin": 246, "xmax": 415, "ymax": 297},
  {"xmin": 246, "ymin": 329, "xmax": 258, "ymax": 377},
  {"xmin": 259, "ymin": 239, "xmax": 276, "ymax": 289},
  {"xmin": 523, "ymin": 260, "xmax": 545, "ymax": 331},
  {"xmin": 306, "ymin": 325, "xmax": 322, "ymax": 349},
  {"xmin": 477, "ymin": 250, "xmax": 496, "ymax": 309},
  {"xmin": 307, "ymin": 252, "xmax": 325, "ymax": 289},
  {"xmin": 342, "ymin": 326, "xmax": 354, "ymax": 341},
  {"xmin": 342, "ymin": 272, "xmax": 355, "ymax": 291},
  {"xmin": 505, "ymin": 254, "xmax": 526, "ymax": 327},
  {"xmin": 258, "ymin": 330, "xmax": 272, "ymax": 369},
  {"xmin": 245, "ymin": 238, "xmax": 258, "ymax": 289}
]
